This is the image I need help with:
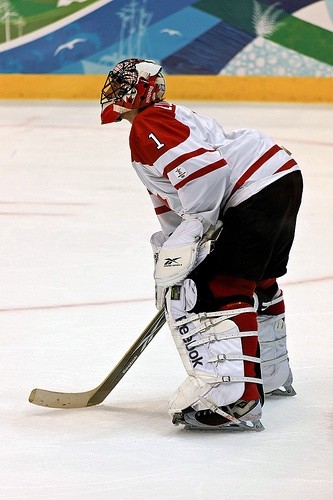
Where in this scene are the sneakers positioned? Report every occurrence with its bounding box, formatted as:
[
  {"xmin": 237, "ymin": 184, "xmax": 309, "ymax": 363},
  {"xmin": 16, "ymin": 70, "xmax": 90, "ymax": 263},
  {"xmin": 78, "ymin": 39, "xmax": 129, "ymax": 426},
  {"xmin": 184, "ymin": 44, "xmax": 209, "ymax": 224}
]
[{"xmin": 172, "ymin": 397, "xmax": 264, "ymax": 433}]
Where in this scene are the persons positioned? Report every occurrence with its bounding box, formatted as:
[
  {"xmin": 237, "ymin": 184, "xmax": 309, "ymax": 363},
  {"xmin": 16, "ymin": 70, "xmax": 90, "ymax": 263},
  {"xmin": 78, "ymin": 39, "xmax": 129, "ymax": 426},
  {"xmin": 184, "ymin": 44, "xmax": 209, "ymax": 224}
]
[{"xmin": 98, "ymin": 58, "xmax": 304, "ymax": 430}]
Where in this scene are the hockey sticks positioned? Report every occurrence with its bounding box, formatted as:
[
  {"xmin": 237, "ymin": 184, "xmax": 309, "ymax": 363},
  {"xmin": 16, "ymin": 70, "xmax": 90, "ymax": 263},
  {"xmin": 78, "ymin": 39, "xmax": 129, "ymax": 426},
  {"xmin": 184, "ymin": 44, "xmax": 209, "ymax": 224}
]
[{"xmin": 28, "ymin": 218, "xmax": 223, "ymax": 408}]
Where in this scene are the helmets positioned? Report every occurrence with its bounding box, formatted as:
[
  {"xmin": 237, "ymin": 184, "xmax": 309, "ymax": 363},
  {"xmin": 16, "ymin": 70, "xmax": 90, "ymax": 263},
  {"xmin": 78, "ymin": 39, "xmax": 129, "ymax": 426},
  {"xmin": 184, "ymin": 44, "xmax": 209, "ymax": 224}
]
[{"xmin": 98, "ymin": 56, "xmax": 166, "ymax": 125}]
